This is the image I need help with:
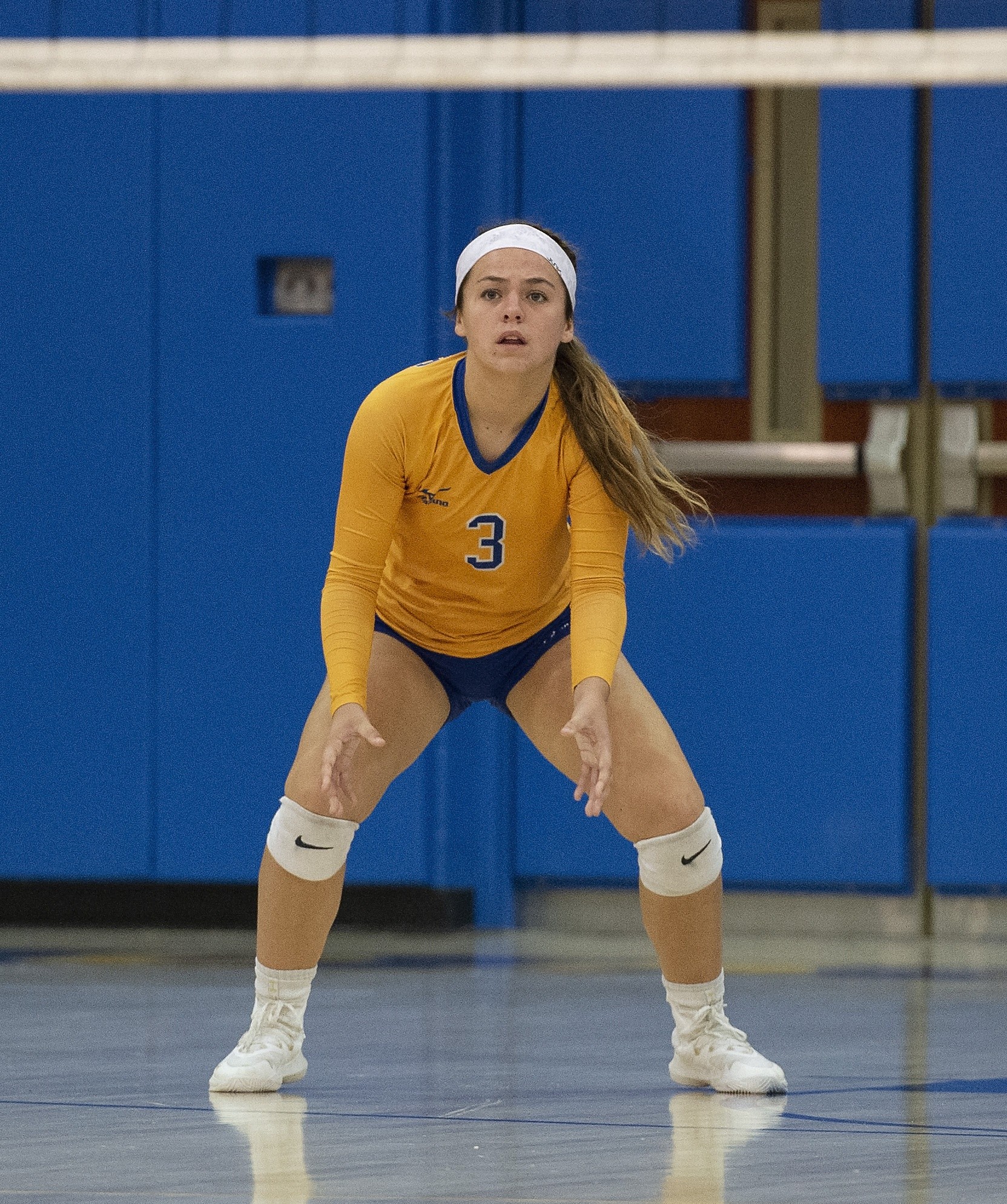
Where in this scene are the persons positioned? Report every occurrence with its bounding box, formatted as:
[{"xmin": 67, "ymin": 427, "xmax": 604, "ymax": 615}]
[{"xmin": 210, "ymin": 221, "xmax": 788, "ymax": 1097}]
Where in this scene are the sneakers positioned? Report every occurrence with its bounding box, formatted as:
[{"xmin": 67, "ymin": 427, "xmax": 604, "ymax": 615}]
[
  {"xmin": 208, "ymin": 998, "xmax": 308, "ymax": 1091},
  {"xmin": 667, "ymin": 996, "xmax": 789, "ymax": 1093}
]
